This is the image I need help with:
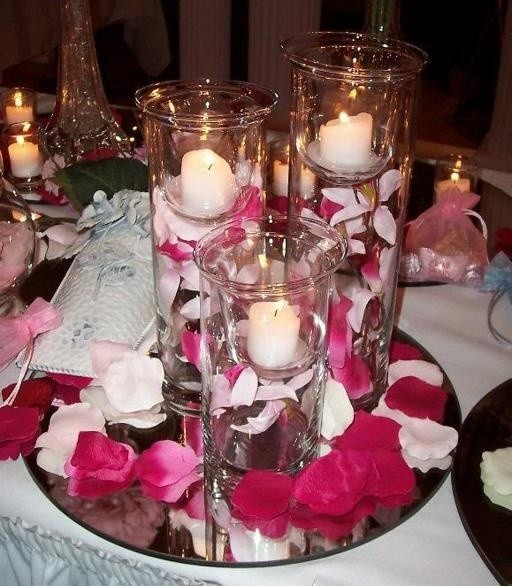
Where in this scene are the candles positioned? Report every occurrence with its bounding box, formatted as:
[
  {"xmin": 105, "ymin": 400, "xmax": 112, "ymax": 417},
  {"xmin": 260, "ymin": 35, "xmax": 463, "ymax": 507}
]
[
  {"xmin": 317, "ymin": 113, "xmax": 374, "ymax": 171},
  {"xmin": 436, "ymin": 172, "xmax": 470, "ymax": 202},
  {"xmin": 3, "ymin": 90, "xmax": 43, "ymax": 179},
  {"xmin": 180, "ymin": 148, "xmax": 236, "ymax": 218},
  {"xmin": 244, "ymin": 297, "xmax": 302, "ymax": 368}
]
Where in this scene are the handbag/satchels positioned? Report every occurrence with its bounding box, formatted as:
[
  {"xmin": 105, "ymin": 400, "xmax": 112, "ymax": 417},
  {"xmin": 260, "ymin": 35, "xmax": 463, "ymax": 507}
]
[
  {"xmin": 54, "ymin": 158, "xmax": 149, "ymax": 214},
  {"xmin": 18, "ymin": 189, "xmax": 163, "ymax": 380}
]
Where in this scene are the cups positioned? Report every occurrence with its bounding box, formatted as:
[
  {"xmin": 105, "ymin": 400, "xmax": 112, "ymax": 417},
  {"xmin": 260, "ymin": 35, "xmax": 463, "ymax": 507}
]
[
  {"xmin": 277, "ymin": 31, "xmax": 431, "ymax": 419},
  {"xmin": 434, "ymin": 154, "xmax": 477, "ymax": 205},
  {"xmin": 193, "ymin": 213, "xmax": 349, "ymax": 511},
  {"xmin": 136, "ymin": 78, "xmax": 278, "ymax": 421},
  {"xmin": 3, "ymin": 85, "xmax": 46, "ymax": 193}
]
